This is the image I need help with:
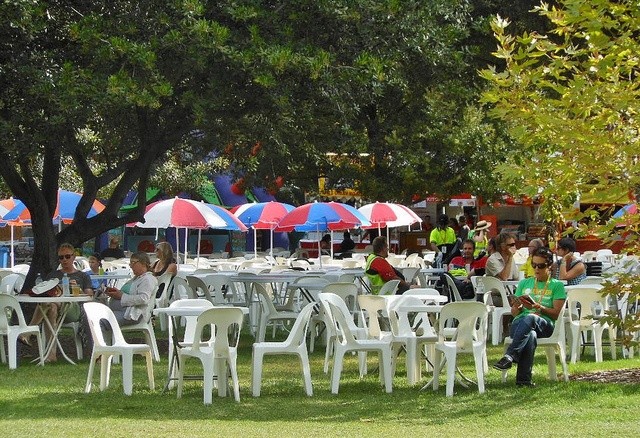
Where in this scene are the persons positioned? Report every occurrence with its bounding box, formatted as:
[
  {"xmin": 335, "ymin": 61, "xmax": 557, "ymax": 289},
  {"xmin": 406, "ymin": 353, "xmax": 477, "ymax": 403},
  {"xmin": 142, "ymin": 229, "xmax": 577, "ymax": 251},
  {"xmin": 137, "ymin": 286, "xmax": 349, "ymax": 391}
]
[
  {"xmin": 148, "ymin": 242, "xmax": 177, "ymax": 307},
  {"xmin": 473, "ymin": 220, "xmax": 492, "ymax": 255},
  {"xmin": 82, "ymin": 254, "xmax": 107, "ymax": 290},
  {"xmin": 555, "ymin": 237, "xmax": 586, "ymax": 286},
  {"xmin": 335, "ymin": 231, "xmax": 355, "ymax": 259},
  {"xmin": 468, "ymin": 237, "xmax": 499, "ymax": 277},
  {"xmin": 101, "ymin": 239, "xmax": 126, "ymax": 257},
  {"xmin": 80, "ymin": 253, "xmax": 159, "ymax": 362},
  {"xmin": 448, "ymin": 240, "xmax": 478, "ymax": 270},
  {"xmin": 517, "ymin": 239, "xmax": 544, "ymax": 277},
  {"xmin": 364, "ymin": 237, "xmax": 411, "ymax": 295},
  {"xmin": 430, "ymin": 214, "xmax": 457, "ymax": 261},
  {"xmin": 493, "ymin": 246, "xmax": 566, "ymax": 386},
  {"xmin": 480, "ymin": 233, "xmax": 519, "ymax": 307},
  {"xmin": 422, "ymin": 215, "xmax": 434, "ymax": 236},
  {"xmin": 18, "ymin": 243, "xmax": 93, "ymax": 362}
]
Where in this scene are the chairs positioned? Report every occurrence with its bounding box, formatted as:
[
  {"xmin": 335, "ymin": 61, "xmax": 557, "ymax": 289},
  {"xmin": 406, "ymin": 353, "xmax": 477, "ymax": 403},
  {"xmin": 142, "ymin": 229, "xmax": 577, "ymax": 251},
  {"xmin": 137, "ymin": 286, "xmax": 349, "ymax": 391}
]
[
  {"xmin": 482, "ymin": 276, "xmax": 512, "ymax": 346},
  {"xmin": 387, "ymin": 295, "xmax": 436, "ymax": 385},
  {"xmin": 254, "ymin": 282, "xmax": 300, "ymax": 343},
  {"xmin": 186, "ymin": 275, "xmax": 218, "ymax": 306},
  {"xmin": 250, "ymin": 302, "xmax": 316, "ymax": 396},
  {"xmin": 0, "ymin": 294, "xmax": 44, "ymax": 369},
  {"xmin": 326, "ymin": 300, "xmax": 393, "ymax": 394},
  {"xmin": 359, "ymin": 295, "xmax": 388, "ymax": 332},
  {"xmin": 205, "ymin": 275, "xmax": 238, "ymax": 305},
  {"xmin": 297, "ymin": 279, "xmax": 331, "ymax": 353},
  {"xmin": 401, "ymin": 288, "xmax": 439, "ymax": 372},
  {"xmin": 83, "ymin": 302, "xmax": 155, "ymax": 396},
  {"xmin": 444, "ymin": 274, "xmax": 476, "ymax": 327},
  {"xmin": 310, "ymin": 284, "xmax": 357, "ymax": 353},
  {"xmin": 72, "ymin": 244, "xmax": 459, "ymax": 274},
  {"xmin": 0, "ymin": 264, "xmax": 30, "ymax": 293},
  {"xmin": 154, "ymin": 272, "xmax": 173, "ymax": 330},
  {"xmin": 502, "ymin": 297, "xmax": 569, "ymax": 383},
  {"xmin": 177, "ymin": 308, "xmax": 243, "ymax": 404},
  {"xmin": 168, "ymin": 299, "xmax": 217, "ymax": 388},
  {"xmin": 377, "ymin": 279, "xmax": 401, "ymax": 295},
  {"xmin": 173, "ymin": 277, "xmax": 193, "ymax": 299},
  {"xmin": 433, "ymin": 302, "xmax": 488, "ymax": 397},
  {"xmin": 112, "ymin": 286, "xmax": 160, "ymax": 364},
  {"xmin": 42, "ymin": 322, "xmax": 83, "ymax": 361},
  {"xmin": 575, "ymin": 250, "xmax": 639, "ymax": 268},
  {"xmin": 567, "ymin": 285, "xmax": 616, "ymax": 364}
]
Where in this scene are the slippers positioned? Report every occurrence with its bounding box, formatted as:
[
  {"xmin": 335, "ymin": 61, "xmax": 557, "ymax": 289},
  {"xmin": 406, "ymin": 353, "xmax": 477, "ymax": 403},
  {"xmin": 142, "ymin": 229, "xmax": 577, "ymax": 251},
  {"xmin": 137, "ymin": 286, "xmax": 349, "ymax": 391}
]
[
  {"xmin": 18, "ymin": 333, "xmax": 33, "ymax": 346},
  {"xmin": 45, "ymin": 358, "xmax": 51, "ymax": 363}
]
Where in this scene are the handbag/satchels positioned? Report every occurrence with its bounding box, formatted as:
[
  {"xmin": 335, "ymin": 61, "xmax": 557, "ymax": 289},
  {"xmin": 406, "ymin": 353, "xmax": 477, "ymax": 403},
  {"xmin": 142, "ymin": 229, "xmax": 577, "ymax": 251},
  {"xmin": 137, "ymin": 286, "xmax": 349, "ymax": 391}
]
[{"xmin": 29, "ymin": 284, "xmax": 63, "ymax": 297}]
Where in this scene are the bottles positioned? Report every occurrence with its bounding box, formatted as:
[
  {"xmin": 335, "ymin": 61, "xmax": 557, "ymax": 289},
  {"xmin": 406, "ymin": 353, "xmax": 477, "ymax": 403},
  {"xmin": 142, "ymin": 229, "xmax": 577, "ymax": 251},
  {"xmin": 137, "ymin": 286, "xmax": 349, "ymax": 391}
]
[
  {"xmin": 62, "ymin": 274, "xmax": 69, "ymax": 297},
  {"xmin": 35, "ymin": 273, "xmax": 43, "ymax": 286}
]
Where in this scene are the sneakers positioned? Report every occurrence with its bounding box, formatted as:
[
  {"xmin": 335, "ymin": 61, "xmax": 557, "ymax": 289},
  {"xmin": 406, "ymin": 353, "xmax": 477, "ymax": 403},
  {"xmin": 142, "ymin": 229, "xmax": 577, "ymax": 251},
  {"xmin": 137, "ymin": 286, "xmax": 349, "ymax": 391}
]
[
  {"xmin": 493, "ymin": 357, "xmax": 512, "ymax": 371},
  {"xmin": 517, "ymin": 381, "xmax": 536, "ymax": 387}
]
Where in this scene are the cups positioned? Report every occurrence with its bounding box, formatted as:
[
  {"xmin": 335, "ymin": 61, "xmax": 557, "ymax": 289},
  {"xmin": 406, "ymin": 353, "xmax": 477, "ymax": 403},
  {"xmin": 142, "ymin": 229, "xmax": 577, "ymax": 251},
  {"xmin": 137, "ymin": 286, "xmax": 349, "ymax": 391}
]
[{"xmin": 72, "ymin": 285, "xmax": 80, "ymax": 296}]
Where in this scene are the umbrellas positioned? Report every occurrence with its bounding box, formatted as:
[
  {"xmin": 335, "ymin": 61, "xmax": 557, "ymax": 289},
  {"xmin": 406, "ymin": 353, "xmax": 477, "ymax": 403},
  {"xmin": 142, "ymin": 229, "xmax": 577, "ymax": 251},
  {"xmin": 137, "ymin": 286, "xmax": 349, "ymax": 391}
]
[
  {"xmin": 228, "ymin": 200, "xmax": 257, "ymax": 257},
  {"xmin": 194, "ymin": 199, "xmax": 249, "ymax": 256},
  {"xmin": 358, "ymin": 201, "xmax": 423, "ymax": 252},
  {"xmin": 128, "ymin": 194, "xmax": 227, "ymax": 257},
  {"xmin": 32, "ymin": 191, "xmax": 104, "ymax": 233},
  {"xmin": 238, "ymin": 201, "xmax": 297, "ymax": 254},
  {"xmin": 0, "ymin": 196, "xmax": 31, "ymax": 266},
  {"xmin": 283, "ymin": 198, "xmax": 372, "ymax": 270}
]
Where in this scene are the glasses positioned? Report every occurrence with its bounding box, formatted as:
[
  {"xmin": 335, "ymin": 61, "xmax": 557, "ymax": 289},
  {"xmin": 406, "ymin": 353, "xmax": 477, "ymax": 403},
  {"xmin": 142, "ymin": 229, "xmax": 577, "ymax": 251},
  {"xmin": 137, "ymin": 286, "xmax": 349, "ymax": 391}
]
[
  {"xmin": 507, "ymin": 243, "xmax": 515, "ymax": 246},
  {"xmin": 557, "ymin": 246, "xmax": 562, "ymax": 249},
  {"xmin": 155, "ymin": 248, "xmax": 159, "ymax": 251},
  {"xmin": 58, "ymin": 254, "xmax": 73, "ymax": 260},
  {"xmin": 129, "ymin": 261, "xmax": 141, "ymax": 265},
  {"xmin": 531, "ymin": 263, "xmax": 551, "ymax": 269}
]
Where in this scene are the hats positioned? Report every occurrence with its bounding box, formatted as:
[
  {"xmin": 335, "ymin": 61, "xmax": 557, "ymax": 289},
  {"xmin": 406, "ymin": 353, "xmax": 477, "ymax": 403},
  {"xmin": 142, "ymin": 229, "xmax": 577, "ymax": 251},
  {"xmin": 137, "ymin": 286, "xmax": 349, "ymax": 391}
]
[
  {"xmin": 439, "ymin": 214, "xmax": 449, "ymax": 224},
  {"xmin": 91, "ymin": 253, "xmax": 101, "ymax": 260},
  {"xmin": 474, "ymin": 220, "xmax": 492, "ymax": 230}
]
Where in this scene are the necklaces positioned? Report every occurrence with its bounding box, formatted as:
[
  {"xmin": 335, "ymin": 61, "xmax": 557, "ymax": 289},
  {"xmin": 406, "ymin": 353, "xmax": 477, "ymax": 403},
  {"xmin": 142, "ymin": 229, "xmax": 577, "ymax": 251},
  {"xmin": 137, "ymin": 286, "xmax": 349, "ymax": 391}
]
[{"xmin": 534, "ymin": 274, "xmax": 550, "ymax": 305}]
